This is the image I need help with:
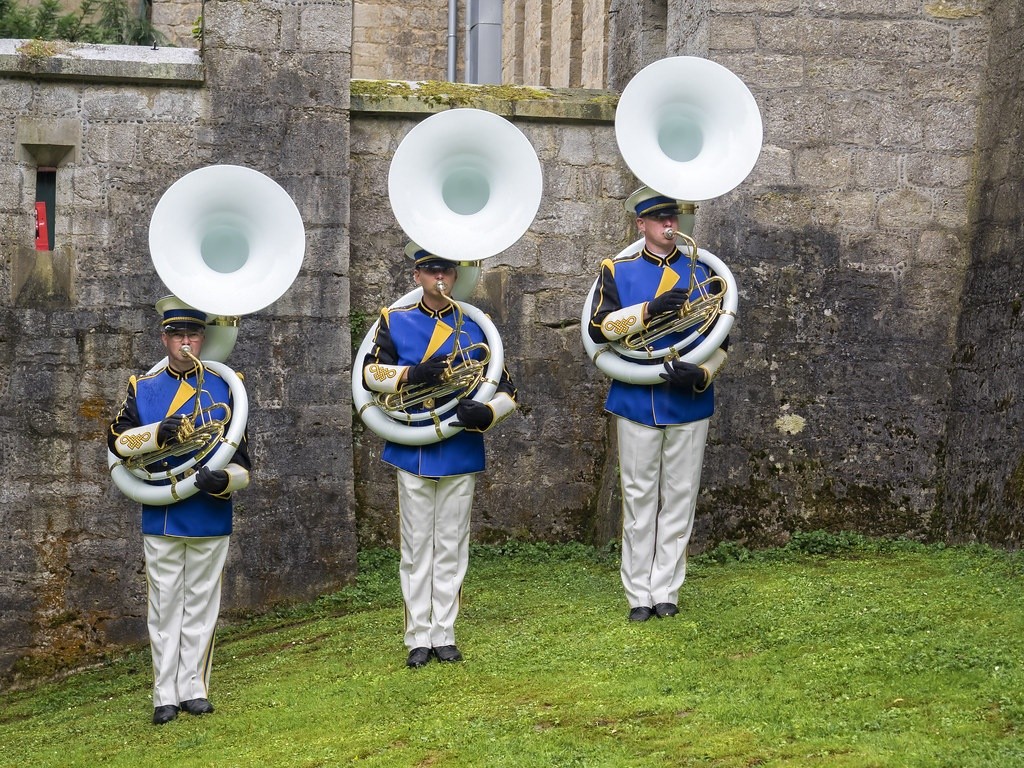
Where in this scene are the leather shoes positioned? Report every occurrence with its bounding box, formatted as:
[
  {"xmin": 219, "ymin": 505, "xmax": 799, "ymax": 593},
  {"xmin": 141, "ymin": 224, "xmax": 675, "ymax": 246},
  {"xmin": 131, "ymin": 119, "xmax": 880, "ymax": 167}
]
[
  {"xmin": 655, "ymin": 602, "xmax": 677, "ymax": 617},
  {"xmin": 154, "ymin": 704, "xmax": 177, "ymax": 725},
  {"xmin": 408, "ymin": 646, "xmax": 431, "ymax": 665},
  {"xmin": 435, "ymin": 646, "xmax": 463, "ymax": 662},
  {"xmin": 629, "ymin": 602, "xmax": 650, "ymax": 621},
  {"xmin": 181, "ymin": 695, "xmax": 213, "ymax": 716}
]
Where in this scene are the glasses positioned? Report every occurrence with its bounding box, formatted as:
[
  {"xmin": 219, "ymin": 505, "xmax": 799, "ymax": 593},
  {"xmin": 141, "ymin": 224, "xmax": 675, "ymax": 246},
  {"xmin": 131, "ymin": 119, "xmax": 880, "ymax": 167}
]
[{"xmin": 166, "ymin": 330, "xmax": 203, "ymax": 343}]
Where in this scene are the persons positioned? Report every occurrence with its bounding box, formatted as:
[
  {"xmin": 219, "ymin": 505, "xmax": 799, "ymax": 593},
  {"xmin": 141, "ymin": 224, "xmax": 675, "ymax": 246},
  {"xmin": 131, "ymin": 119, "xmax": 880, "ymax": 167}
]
[
  {"xmin": 590, "ymin": 188, "xmax": 730, "ymax": 622},
  {"xmin": 362, "ymin": 242, "xmax": 518, "ymax": 667},
  {"xmin": 106, "ymin": 295, "xmax": 251, "ymax": 725}
]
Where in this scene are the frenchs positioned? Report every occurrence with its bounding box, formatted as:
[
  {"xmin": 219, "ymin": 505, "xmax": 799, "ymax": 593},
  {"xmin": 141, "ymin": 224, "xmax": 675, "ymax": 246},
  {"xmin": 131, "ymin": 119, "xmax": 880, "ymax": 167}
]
[
  {"xmin": 107, "ymin": 164, "xmax": 306, "ymax": 506},
  {"xmin": 580, "ymin": 54, "xmax": 764, "ymax": 386},
  {"xmin": 349, "ymin": 107, "xmax": 543, "ymax": 446}
]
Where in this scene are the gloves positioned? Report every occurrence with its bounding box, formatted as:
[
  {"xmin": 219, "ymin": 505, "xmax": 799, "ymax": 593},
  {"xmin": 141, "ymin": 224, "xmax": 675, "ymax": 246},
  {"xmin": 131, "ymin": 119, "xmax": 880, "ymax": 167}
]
[
  {"xmin": 658, "ymin": 356, "xmax": 704, "ymax": 386},
  {"xmin": 448, "ymin": 397, "xmax": 492, "ymax": 430},
  {"xmin": 646, "ymin": 286, "xmax": 688, "ymax": 316},
  {"xmin": 157, "ymin": 414, "xmax": 182, "ymax": 439},
  {"xmin": 194, "ymin": 465, "xmax": 229, "ymax": 493},
  {"xmin": 409, "ymin": 356, "xmax": 450, "ymax": 387}
]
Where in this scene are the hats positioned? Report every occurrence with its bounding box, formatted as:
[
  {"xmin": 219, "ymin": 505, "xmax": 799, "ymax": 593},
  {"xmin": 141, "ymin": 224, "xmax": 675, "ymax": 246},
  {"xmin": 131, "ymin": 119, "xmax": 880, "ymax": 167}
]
[
  {"xmin": 156, "ymin": 293, "xmax": 207, "ymax": 333},
  {"xmin": 623, "ymin": 181, "xmax": 679, "ymax": 220},
  {"xmin": 404, "ymin": 240, "xmax": 462, "ymax": 272}
]
